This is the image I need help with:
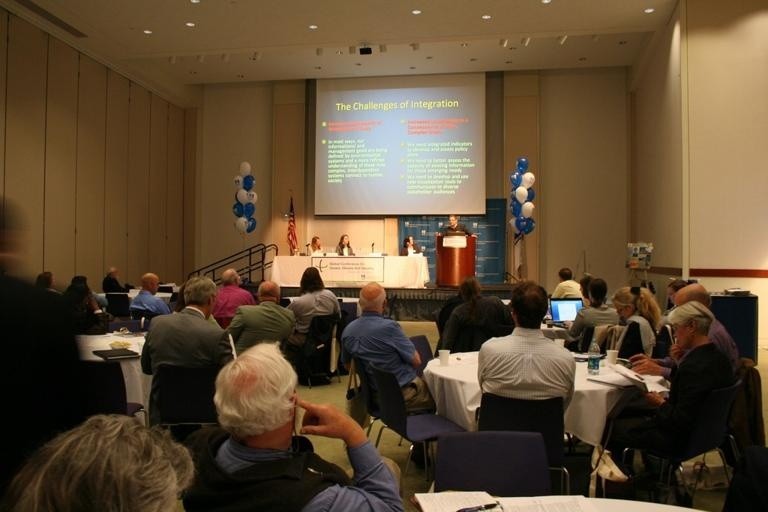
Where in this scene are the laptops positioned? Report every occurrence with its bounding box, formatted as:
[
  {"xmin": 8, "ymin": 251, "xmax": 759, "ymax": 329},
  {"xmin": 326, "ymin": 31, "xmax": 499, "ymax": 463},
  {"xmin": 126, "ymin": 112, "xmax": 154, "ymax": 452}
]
[{"xmin": 549, "ymin": 297, "xmax": 583, "ymax": 329}]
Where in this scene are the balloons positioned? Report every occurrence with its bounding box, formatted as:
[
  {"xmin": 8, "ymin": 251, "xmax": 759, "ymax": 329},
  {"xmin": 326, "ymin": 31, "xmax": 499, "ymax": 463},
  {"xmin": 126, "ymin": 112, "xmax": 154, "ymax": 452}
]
[
  {"xmin": 232, "ymin": 162, "xmax": 257, "ymax": 235},
  {"xmin": 508, "ymin": 156, "xmax": 536, "ymax": 234}
]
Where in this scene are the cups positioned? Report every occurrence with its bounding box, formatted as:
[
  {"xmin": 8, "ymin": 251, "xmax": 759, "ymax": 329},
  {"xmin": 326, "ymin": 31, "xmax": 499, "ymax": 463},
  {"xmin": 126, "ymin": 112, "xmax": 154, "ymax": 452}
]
[
  {"xmin": 555, "ymin": 339, "xmax": 566, "ymax": 347},
  {"xmin": 438, "ymin": 349, "xmax": 451, "ymax": 366},
  {"xmin": 606, "ymin": 347, "xmax": 621, "ymax": 366},
  {"xmin": 547, "ymin": 318, "xmax": 553, "ymax": 328}
]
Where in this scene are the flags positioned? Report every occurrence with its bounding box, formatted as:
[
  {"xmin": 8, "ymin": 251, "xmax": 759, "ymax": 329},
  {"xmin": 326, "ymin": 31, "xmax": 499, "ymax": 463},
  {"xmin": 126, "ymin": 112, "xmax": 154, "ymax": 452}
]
[{"xmin": 286, "ymin": 196, "xmax": 298, "ymax": 256}]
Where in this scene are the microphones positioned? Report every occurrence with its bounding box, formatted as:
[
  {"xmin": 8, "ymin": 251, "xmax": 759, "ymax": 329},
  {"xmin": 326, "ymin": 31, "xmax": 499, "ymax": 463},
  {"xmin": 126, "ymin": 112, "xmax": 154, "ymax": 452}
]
[
  {"xmin": 306, "ymin": 243, "xmax": 310, "ymax": 246},
  {"xmin": 437, "ymin": 221, "xmax": 444, "ymax": 233},
  {"xmin": 371, "ymin": 243, "xmax": 374, "ymax": 246}
]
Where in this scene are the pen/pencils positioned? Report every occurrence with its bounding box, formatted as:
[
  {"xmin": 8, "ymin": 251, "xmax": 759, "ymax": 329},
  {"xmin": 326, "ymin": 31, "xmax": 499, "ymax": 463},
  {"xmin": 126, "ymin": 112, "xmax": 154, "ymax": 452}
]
[{"xmin": 456, "ymin": 504, "xmax": 497, "ymax": 512}]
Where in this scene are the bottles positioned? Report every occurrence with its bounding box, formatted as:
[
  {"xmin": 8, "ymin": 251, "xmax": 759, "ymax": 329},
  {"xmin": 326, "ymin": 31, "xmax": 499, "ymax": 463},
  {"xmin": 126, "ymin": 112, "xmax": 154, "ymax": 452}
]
[
  {"xmin": 294, "ymin": 248, "xmax": 299, "ymax": 257},
  {"xmin": 586, "ymin": 339, "xmax": 601, "ymax": 374}
]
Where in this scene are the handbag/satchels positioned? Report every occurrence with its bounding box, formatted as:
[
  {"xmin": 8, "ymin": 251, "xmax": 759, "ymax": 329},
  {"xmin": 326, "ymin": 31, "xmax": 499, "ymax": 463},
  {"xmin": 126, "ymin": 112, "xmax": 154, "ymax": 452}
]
[{"xmin": 344, "ymin": 358, "xmax": 371, "ymax": 431}]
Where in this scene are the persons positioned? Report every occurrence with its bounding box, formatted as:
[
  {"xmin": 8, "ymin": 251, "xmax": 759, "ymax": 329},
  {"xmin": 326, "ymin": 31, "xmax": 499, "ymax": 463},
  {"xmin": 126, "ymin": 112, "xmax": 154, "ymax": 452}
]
[
  {"xmin": 308, "ymin": 237, "xmax": 322, "ymax": 256},
  {"xmin": 0, "ymin": 198, "xmax": 84, "ymax": 495},
  {"xmin": 400, "ymin": 236, "xmax": 421, "ymax": 256},
  {"xmin": 334, "ymin": 234, "xmax": 354, "ymax": 256},
  {"xmin": 434, "ymin": 267, "xmax": 745, "ymax": 504},
  {"xmin": 4, "ymin": 414, "xmax": 197, "ymax": 510},
  {"xmin": 138, "ymin": 268, "xmax": 438, "ymax": 510},
  {"xmin": 435, "ymin": 216, "xmax": 477, "ymax": 238},
  {"xmin": 35, "ymin": 268, "xmax": 172, "ymax": 331}
]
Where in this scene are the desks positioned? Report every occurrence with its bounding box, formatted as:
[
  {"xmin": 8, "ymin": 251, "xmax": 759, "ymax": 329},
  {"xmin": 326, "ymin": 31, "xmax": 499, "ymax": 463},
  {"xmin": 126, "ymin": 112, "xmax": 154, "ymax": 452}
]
[{"xmin": 270, "ymin": 254, "xmax": 431, "ymax": 289}]
